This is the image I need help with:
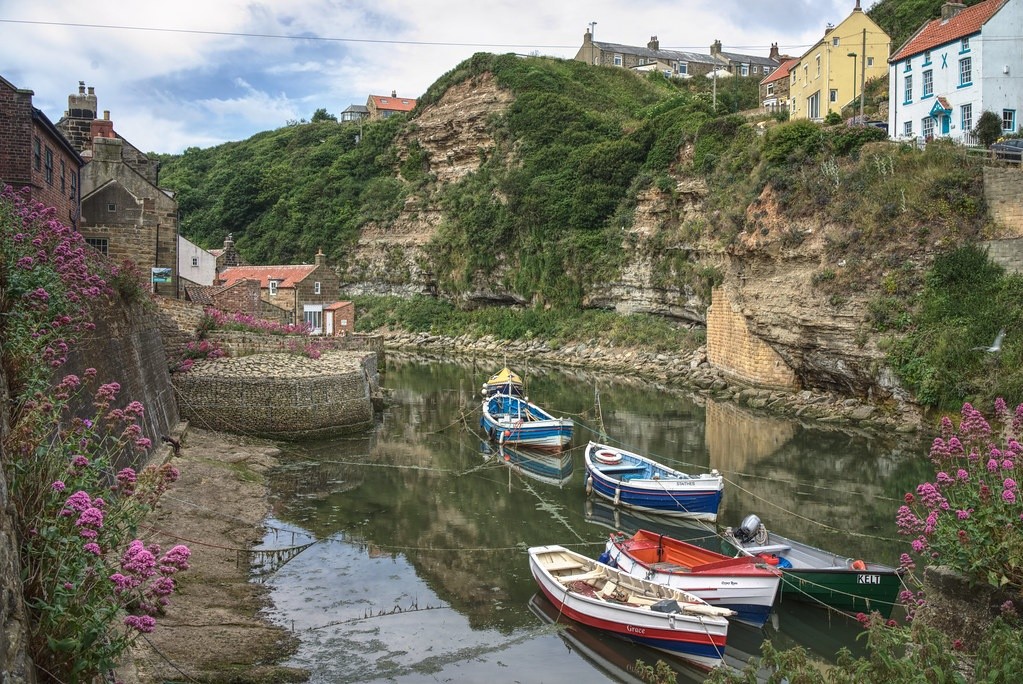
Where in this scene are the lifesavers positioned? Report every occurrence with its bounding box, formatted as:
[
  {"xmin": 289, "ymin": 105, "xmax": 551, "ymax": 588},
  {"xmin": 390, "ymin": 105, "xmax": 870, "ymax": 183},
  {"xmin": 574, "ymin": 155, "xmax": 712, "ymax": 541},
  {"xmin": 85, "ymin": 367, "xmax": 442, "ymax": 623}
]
[{"xmin": 596, "ymin": 450, "xmax": 622, "ymax": 464}]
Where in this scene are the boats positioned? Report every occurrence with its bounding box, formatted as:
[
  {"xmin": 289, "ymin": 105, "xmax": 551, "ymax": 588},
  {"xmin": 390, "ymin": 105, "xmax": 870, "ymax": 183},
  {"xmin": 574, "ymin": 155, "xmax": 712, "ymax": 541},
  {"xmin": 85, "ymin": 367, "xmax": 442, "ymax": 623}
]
[
  {"xmin": 527, "ymin": 543, "xmax": 739, "ymax": 673},
  {"xmin": 593, "ymin": 528, "xmax": 783, "ymax": 628},
  {"xmin": 481, "ymin": 391, "xmax": 575, "ymax": 451},
  {"xmin": 481, "ymin": 356, "xmax": 526, "ymax": 398},
  {"xmin": 584, "ymin": 440, "xmax": 725, "ymax": 524},
  {"xmin": 723, "ymin": 514, "xmax": 911, "ymax": 621}
]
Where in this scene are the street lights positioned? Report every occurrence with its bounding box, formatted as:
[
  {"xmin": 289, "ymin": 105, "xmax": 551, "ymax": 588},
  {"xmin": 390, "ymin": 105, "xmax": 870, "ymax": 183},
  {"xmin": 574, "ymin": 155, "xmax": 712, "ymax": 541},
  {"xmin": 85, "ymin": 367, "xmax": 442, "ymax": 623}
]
[{"xmin": 848, "ymin": 52, "xmax": 857, "ymax": 125}]
